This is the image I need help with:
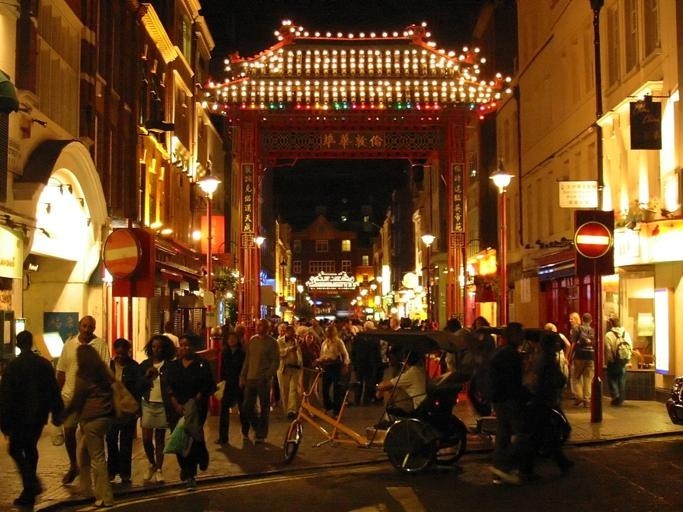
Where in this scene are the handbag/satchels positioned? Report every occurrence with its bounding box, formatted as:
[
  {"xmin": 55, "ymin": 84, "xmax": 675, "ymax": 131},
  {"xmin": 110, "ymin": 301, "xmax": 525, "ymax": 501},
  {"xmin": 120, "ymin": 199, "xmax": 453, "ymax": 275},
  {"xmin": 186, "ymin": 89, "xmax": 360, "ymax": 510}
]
[
  {"xmin": 101, "ymin": 361, "xmax": 140, "ymax": 420},
  {"xmin": 161, "ymin": 415, "xmax": 196, "ymax": 458}
]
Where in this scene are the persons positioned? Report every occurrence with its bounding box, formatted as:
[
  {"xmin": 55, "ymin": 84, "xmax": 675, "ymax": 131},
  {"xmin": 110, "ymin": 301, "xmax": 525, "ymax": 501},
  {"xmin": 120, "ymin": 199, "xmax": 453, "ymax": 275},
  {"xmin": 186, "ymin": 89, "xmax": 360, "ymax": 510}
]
[
  {"xmin": 373, "ymin": 351, "xmax": 426, "ymax": 429},
  {"xmin": 214, "ymin": 316, "xmax": 388, "ymax": 444},
  {"xmin": 1, "ymin": 314, "xmax": 212, "ymax": 511},
  {"xmin": 389, "ymin": 315, "xmax": 439, "ymax": 332},
  {"xmin": 545, "ymin": 315, "xmax": 632, "ymax": 408},
  {"xmin": 449, "ymin": 317, "xmax": 543, "ymax": 484}
]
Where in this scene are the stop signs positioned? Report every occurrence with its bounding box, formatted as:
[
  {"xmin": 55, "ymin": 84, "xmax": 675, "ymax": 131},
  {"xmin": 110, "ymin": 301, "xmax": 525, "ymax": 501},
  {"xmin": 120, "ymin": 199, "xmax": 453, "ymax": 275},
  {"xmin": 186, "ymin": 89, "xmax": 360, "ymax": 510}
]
[
  {"xmin": 99, "ymin": 230, "xmax": 143, "ymax": 280},
  {"xmin": 571, "ymin": 218, "xmax": 613, "ymax": 261}
]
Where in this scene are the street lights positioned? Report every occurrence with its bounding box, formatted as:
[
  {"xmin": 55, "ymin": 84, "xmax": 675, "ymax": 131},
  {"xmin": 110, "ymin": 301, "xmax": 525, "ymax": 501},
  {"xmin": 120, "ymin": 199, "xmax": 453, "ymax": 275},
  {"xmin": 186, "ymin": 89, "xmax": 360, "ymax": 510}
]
[
  {"xmin": 418, "ymin": 231, "xmax": 437, "ymax": 322},
  {"xmin": 460, "ymin": 238, "xmax": 495, "ymax": 329},
  {"xmin": 487, "ymin": 160, "xmax": 516, "ymax": 341},
  {"xmin": 348, "ymin": 274, "xmax": 382, "ymax": 324},
  {"xmin": 213, "ymin": 240, "xmax": 243, "ymax": 324},
  {"xmin": 194, "ymin": 173, "xmax": 222, "ymax": 354},
  {"xmin": 279, "ymin": 258, "xmax": 287, "ymax": 322},
  {"xmin": 251, "ymin": 227, "xmax": 266, "ymax": 322},
  {"xmin": 288, "ymin": 273, "xmax": 315, "ymax": 317}
]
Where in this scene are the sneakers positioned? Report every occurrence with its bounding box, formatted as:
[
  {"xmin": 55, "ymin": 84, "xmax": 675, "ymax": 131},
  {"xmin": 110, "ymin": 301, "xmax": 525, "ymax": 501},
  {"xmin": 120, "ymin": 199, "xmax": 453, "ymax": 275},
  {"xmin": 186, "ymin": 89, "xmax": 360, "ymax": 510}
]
[
  {"xmin": 95, "ymin": 499, "xmax": 113, "ymax": 508},
  {"xmin": 120, "ymin": 475, "xmax": 131, "ymax": 483},
  {"xmin": 143, "ymin": 467, "xmax": 164, "ymax": 483},
  {"xmin": 214, "ymin": 438, "xmax": 228, "ymax": 444},
  {"xmin": 243, "ymin": 434, "xmax": 265, "ymax": 444},
  {"xmin": 287, "ymin": 409, "xmax": 297, "ymax": 420},
  {"xmin": 186, "ymin": 477, "xmax": 197, "ymax": 491},
  {"xmin": 373, "ymin": 420, "xmax": 391, "ymax": 430},
  {"xmin": 610, "ymin": 395, "xmax": 626, "ymax": 406},
  {"xmin": 61, "ymin": 465, "xmax": 80, "ymax": 484},
  {"xmin": 108, "ymin": 471, "xmax": 117, "ymax": 481},
  {"xmin": 573, "ymin": 399, "xmax": 592, "ymax": 408},
  {"xmin": 490, "ymin": 466, "xmax": 525, "ymax": 485}
]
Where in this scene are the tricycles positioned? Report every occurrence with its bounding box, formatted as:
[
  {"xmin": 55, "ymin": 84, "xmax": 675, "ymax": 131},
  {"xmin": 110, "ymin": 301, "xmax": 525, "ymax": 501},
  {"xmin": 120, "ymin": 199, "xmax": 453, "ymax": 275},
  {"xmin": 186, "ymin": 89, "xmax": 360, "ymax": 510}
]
[
  {"xmin": 459, "ymin": 323, "xmax": 571, "ymax": 446},
  {"xmin": 276, "ymin": 328, "xmax": 471, "ymax": 476}
]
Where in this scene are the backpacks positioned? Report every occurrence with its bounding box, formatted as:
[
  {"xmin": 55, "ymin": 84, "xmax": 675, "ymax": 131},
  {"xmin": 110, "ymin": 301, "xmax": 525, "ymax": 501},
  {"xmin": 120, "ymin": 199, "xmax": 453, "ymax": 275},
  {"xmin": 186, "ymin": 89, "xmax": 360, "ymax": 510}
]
[
  {"xmin": 575, "ymin": 326, "xmax": 595, "ymax": 355},
  {"xmin": 608, "ymin": 328, "xmax": 632, "ymax": 362}
]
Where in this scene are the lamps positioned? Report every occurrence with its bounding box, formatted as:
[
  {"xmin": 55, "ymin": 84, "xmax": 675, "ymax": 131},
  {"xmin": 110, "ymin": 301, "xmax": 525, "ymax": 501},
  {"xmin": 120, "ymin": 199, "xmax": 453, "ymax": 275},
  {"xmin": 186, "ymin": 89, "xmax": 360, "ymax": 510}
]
[{"xmin": 29, "ymin": 178, "xmax": 93, "ymax": 240}]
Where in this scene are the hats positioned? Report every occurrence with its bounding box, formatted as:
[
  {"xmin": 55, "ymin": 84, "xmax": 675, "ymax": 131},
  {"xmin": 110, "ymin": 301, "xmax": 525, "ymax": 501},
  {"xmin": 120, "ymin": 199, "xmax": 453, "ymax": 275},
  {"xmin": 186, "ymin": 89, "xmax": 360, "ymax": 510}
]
[{"xmin": 364, "ymin": 321, "xmax": 378, "ymax": 330}]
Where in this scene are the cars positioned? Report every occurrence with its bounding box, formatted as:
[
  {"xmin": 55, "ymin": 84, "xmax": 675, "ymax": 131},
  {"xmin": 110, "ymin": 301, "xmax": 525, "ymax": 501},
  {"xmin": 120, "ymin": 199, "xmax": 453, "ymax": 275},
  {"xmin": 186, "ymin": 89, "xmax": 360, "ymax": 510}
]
[{"xmin": 664, "ymin": 373, "xmax": 682, "ymax": 426}]
